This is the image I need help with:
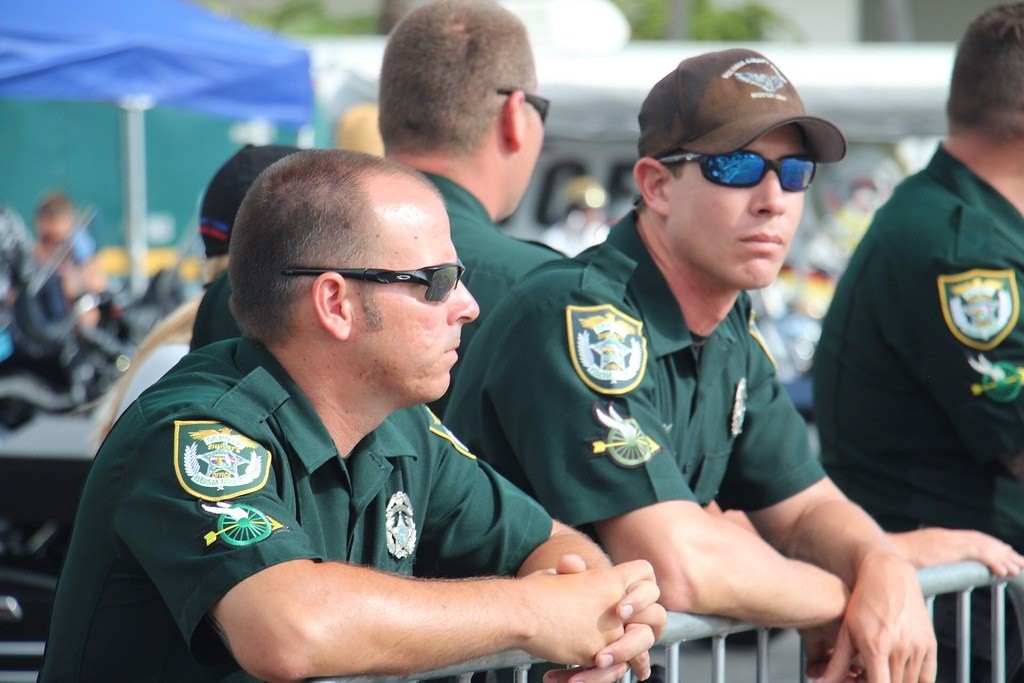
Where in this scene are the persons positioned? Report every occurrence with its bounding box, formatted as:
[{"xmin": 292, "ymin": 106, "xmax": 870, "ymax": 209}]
[{"xmin": 0, "ymin": 0, "xmax": 1024, "ymax": 683}]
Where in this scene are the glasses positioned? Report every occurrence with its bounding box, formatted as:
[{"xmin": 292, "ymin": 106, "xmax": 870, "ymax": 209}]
[
  {"xmin": 281, "ymin": 260, "xmax": 466, "ymax": 304},
  {"xmin": 658, "ymin": 150, "xmax": 817, "ymax": 191}
]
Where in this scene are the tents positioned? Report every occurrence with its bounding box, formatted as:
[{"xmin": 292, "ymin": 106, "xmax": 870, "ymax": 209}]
[{"xmin": 0, "ymin": 0, "xmax": 316, "ymax": 306}]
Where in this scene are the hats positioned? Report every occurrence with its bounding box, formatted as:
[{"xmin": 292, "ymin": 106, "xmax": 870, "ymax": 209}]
[
  {"xmin": 637, "ymin": 49, "xmax": 847, "ymax": 163},
  {"xmin": 200, "ymin": 144, "xmax": 304, "ymax": 257}
]
